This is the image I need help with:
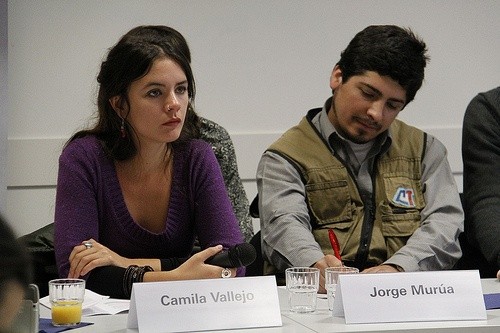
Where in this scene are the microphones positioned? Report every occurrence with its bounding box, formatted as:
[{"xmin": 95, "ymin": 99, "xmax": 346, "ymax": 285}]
[{"xmin": 204, "ymin": 242, "xmax": 257, "ymax": 268}]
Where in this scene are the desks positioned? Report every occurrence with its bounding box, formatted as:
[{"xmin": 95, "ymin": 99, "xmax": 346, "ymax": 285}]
[{"xmin": 39, "ymin": 279, "xmax": 500, "ymax": 333}]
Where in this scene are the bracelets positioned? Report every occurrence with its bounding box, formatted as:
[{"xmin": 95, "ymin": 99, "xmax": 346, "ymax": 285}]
[
  {"xmin": 123, "ymin": 264, "xmax": 155, "ymax": 300},
  {"xmin": 387, "ymin": 263, "xmax": 404, "ymax": 271}
]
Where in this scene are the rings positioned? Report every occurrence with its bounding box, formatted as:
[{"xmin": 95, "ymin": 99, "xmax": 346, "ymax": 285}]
[
  {"xmin": 222, "ymin": 268, "xmax": 232, "ymax": 278},
  {"xmin": 84, "ymin": 242, "xmax": 93, "ymax": 250}
]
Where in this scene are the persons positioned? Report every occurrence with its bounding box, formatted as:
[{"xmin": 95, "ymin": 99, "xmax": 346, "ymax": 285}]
[
  {"xmin": 0, "ymin": 216, "xmax": 30, "ymax": 333},
  {"xmin": 462, "ymin": 85, "xmax": 500, "ymax": 282},
  {"xmin": 123, "ymin": 24, "xmax": 254, "ymax": 259},
  {"xmin": 256, "ymin": 25, "xmax": 465, "ymax": 295},
  {"xmin": 53, "ymin": 37, "xmax": 247, "ymax": 301}
]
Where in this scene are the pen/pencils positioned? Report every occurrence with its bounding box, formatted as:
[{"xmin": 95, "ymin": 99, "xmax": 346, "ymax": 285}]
[{"xmin": 327, "ymin": 228, "xmax": 343, "ymax": 260}]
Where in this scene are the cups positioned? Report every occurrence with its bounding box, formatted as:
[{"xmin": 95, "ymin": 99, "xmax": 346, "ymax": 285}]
[
  {"xmin": 324, "ymin": 267, "xmax": 359, "ymax": 311},
  {"xmin": 285, "ymin": 267, "xmax": 320, "ymax": 313},
  {"xmin": 48, "ymin": 278, "xmax": 85, "ymax": 327}
]
